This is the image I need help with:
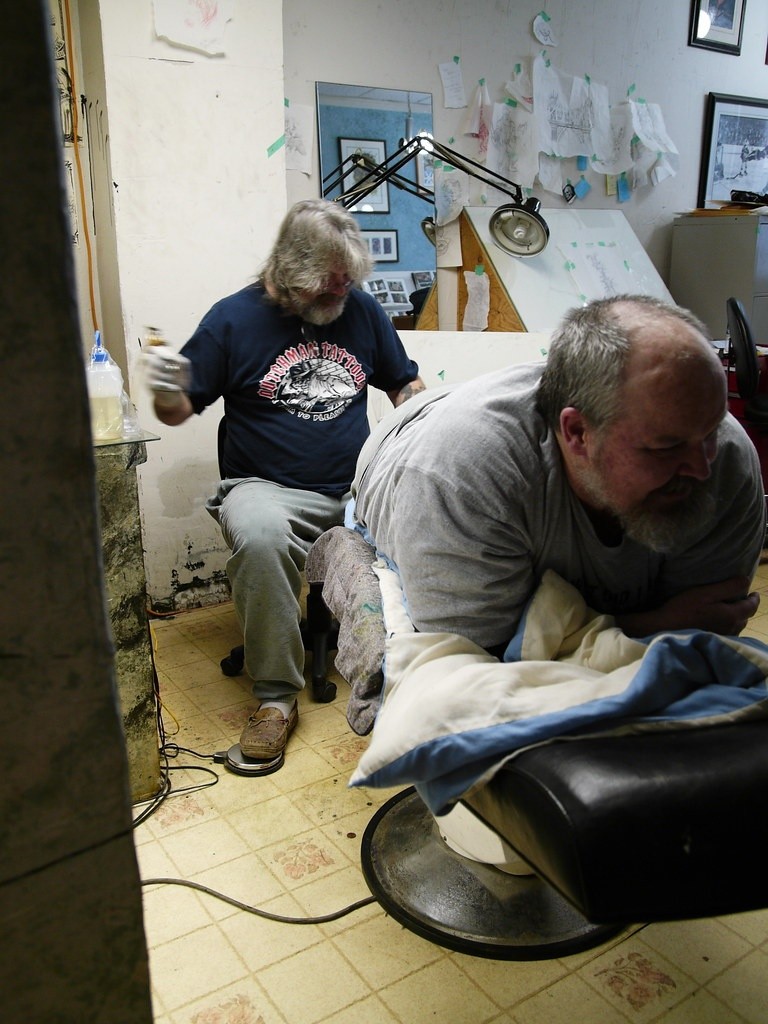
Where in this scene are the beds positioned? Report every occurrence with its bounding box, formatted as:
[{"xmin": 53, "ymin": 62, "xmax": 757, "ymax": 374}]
[{"xmin": 304, "ymin": 527, "xmax": 767, "ymax": 963}]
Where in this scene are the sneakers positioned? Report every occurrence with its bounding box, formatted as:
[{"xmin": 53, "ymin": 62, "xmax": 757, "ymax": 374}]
[{"xmin": 240, "ymin": 699, "xmax": 299, "ymax": 759}]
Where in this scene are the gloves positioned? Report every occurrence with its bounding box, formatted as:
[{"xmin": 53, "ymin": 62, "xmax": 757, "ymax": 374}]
[{"xmin": 136, "ymin": 346, "xmax": 195, "ymax": 408}]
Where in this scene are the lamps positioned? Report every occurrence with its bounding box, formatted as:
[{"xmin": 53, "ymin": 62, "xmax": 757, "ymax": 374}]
[
  {"xmin": 398, "ymin": 92, "xmax": 433, "ymax": 156},
  {"xmin": 319, "ymin": 155, "xmax": 438, "ymax": 244},
  {"xmin": 334, "ymin": 137, "xmax": 550, "ymax": 257}
]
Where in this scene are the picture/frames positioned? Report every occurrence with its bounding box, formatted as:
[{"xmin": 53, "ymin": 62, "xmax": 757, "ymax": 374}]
[
  {"xmin": 687, "ymin": 0, "xmax": 747, "ymax": 57},
  {"xmin": 338, "ymin": 139, "xmax": 390, "ymax": 214},
  {"xmin": 695, "ymin": 92, "xmax": 768, "ymax": 213},
  {"xmin": 357, "ymin": 229, "xmax": 399, "ymax": 263}
]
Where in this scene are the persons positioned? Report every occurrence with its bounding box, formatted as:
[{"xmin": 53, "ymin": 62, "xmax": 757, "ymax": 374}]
[
  {"xmin": 739, "ymin": 144, "xmax": 749, "ymax": 176},
  {"xmin": 140, "ymin": 198, "xmax": 426, "ymax": 764},
  {"xmin": 343, "ymin": 293, "xmax": 765, "ymax": 660}
]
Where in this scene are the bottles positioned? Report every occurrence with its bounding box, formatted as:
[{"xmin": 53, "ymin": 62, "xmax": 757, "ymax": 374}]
[{"xmin": 85, "ymin": 330, "xmax": 123, "ymax": 441}]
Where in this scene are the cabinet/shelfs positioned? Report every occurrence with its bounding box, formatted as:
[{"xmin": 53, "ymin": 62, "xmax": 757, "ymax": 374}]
[{"xmin": 670, "ymin": 216, "xmax": 768, "ymax": 346}]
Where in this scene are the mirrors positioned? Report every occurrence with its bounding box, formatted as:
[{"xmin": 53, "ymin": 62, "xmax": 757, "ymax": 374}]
[{"xmin": 315, "ymin": 80, "xmax": 438, "ymax": 272}]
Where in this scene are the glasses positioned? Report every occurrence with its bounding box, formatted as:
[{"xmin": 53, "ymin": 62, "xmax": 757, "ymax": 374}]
[{"xmin": 322, "ymin": 280, "xmax": 354, "ymax": 293}]
